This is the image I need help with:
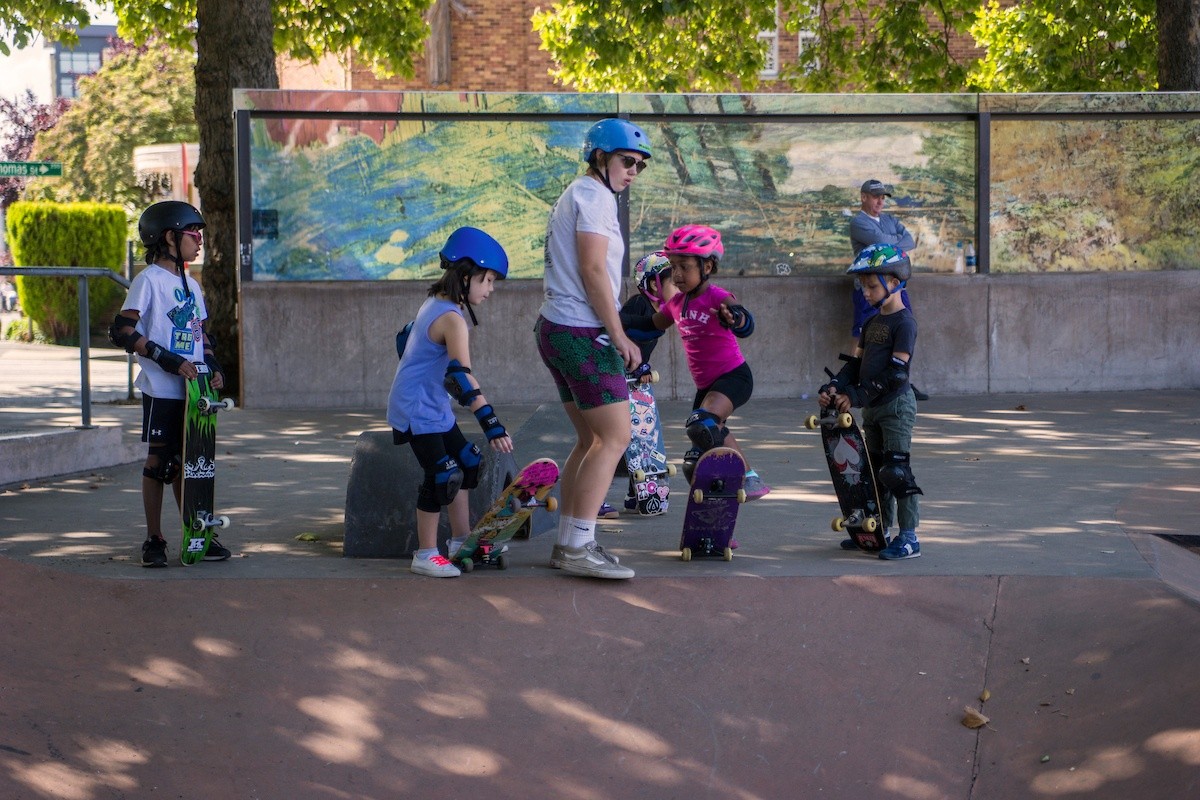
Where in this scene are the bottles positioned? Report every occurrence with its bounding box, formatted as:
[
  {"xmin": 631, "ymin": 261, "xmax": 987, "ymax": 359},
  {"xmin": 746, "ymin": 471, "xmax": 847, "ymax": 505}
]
[
  {"xmin": 965, "ymin": 240, "xmax": 976, "ymax": 274},
  {"xmin": 953, "ymin": 240, "xmax": 965, "ymax": 274}
]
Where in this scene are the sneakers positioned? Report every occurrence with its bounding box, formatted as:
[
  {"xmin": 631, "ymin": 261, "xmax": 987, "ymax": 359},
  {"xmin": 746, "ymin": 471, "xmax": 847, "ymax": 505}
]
[
  {"xmin": 597, "ymin": 503, "xmax": 619, "ymax": 518},
  {"xmin": 910, "ymin": 383, "xmax": 929, "ymax": 401},
  {"xmin": 202, "ymin": 540, "xmax": 231, "ymax": 561},
  {"xmin": 731, "ymin": 534, "xmax": 738, "ymax": 549},
  {"xmin": 840, "ymin": 531, "xmax": 890, "ymax": 551},
  {"xmin": 878, "ymin": 536, "xmax": 920, "ymax": 560},
  {"xmin": 447, "ymin": 540, "xmax": 509, "ymax": 559},
  {"xmin": 142, "ymin": 534, "xmax": 168, "ymax": 568},
  {"xmin": 744, "ymin": 476, "xmax": 769, "ymax": 502},
  {"xmin": 410, "ymin": 550, "xmax": 461, "ymax": 577},
  {"xmin": 551, "ymin": 540, "xmax": 635, "ymax": 578}
]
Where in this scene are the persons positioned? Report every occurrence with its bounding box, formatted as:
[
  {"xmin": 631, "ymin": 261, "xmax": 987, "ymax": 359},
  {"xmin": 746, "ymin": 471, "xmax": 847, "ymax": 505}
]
[
  {"xmin": 848, "ymin": 181, "xmax": 929, "ymax": 401},
  {"xmin": 816, "ymin": 244, "xmax": 921, "ymax": 559},
  {"xmin": 385, "ymin": 226, "xmax": 514, "ymax": 578},
  {"xmin": 111, "ymin": 201, "xmax": 230, "ymax": 566},
  {"xmin": 528, "ymin": 118, "xmax": 653, "ymax": 577},
  {"xmin": 618, "ymin": 223, "xmax": 769, "ymax": 512}
]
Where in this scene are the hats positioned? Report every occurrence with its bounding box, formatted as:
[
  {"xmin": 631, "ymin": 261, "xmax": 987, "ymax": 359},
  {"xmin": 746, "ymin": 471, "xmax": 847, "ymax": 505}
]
[{"xmin": 862, "ymin": 180, "xmax": 891, "ymax": 198}]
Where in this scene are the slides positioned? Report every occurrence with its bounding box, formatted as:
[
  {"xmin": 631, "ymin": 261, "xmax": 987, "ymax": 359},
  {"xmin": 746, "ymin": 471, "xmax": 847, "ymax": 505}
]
[{"xmin": 679, "ymin": 446, "xmax": 747, "ymax": 565}]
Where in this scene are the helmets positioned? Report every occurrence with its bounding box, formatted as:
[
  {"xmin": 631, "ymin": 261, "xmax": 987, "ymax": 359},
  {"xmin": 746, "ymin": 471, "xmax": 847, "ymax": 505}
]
[
  {"xmin": 847, "ymin": 242, "xmax": 912, "ymax": 280},
  {"xmin": 584, "ymin": 118, "xmax": 652, "ymax": 163},
  {"xmin": 440, "ymin": 227, "xmax": 508, "ymax": 280},
  {"xmin": 664, "ymin": 225, "xmax": 724, "ymax": 260},
  {"xmin": 138, "ymin": 201, "xmax": 207, "ymax": 249},
  {"xmin": 634, "ymin": 250, "xmax": 673, "ymax": 295}
]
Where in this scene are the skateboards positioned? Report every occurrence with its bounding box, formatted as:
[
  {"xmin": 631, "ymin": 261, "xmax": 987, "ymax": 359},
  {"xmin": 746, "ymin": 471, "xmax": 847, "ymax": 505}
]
[
  {"xmin": 448, "ymin": 457, "xmax": 561, "ymax": 572},
  {"xmin": 801, "ymin": 390, "xmax": 892, "ymax": 558},
  {"xmin": 616, "ymin": 357, "xmax": 678, "ymax": 518},
  {"xmin": 178, "ymin": 360, "xmax": 235, "ymax": 568}
]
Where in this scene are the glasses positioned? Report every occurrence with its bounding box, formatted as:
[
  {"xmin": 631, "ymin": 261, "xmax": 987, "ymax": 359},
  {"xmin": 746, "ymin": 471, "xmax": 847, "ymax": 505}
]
[
  {"xmin": 615, "ymin": 153, "xmax": 647, "ymax": 174},
  {"xmin": 181, "ymin": 231, "xmax": 202, "ymax": 242}
]
